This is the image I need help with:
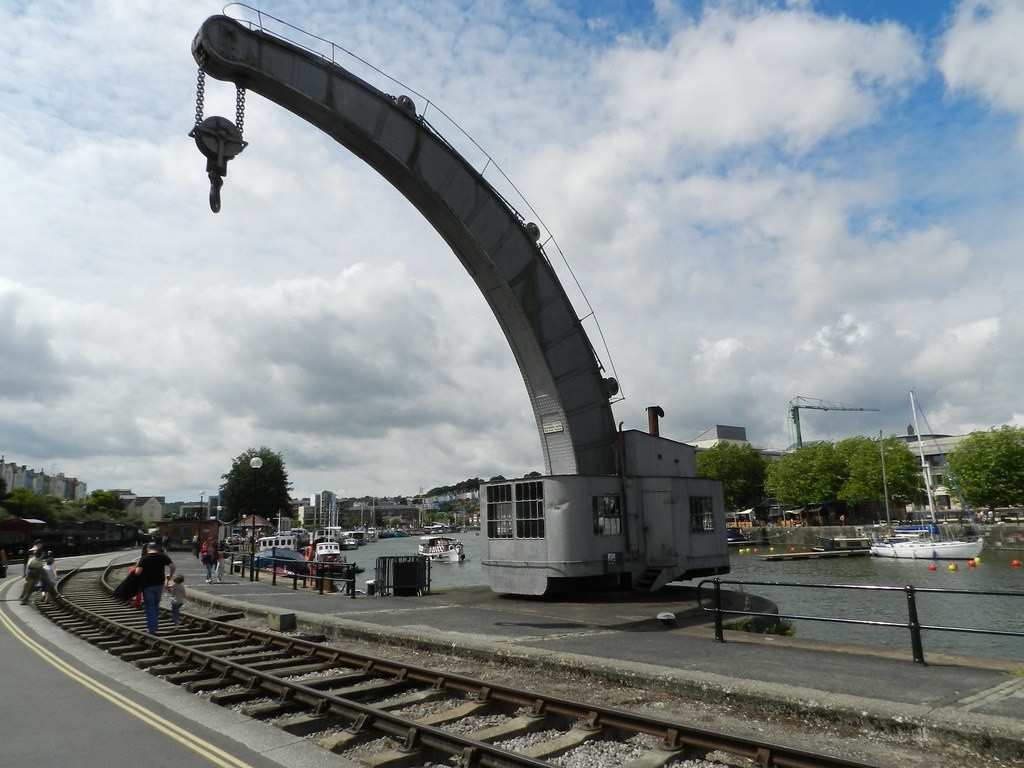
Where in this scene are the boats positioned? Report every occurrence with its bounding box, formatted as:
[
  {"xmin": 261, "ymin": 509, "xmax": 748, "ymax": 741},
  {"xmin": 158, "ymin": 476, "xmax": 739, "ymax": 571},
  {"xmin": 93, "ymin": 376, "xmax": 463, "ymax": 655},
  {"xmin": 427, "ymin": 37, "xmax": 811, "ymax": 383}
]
[
  {"xmin": 724, "ymin": 526, "xmax": 760, "ymax": 547},
  {"xmin": 241, "ymin": 522, "xmax": 467, "ymax": 579},
  {"xmin": 810, "ymin": 533, "xmax": 886, "ymax": 551}
]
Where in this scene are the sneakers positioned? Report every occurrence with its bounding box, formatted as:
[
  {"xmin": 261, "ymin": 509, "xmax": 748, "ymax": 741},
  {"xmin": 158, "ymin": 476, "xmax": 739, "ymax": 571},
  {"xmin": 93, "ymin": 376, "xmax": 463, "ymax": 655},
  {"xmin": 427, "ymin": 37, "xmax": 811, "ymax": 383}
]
[
  {"xmin": 209, "ymin": 578, "xmax": 212, "ymax": 582},
  {"xmin": 206, "ymin": 579, "xmax": 210, "ymax": 583},
  {"xmin": 218, "ymin": 578, "xmax": 222, "ymax": 582}
]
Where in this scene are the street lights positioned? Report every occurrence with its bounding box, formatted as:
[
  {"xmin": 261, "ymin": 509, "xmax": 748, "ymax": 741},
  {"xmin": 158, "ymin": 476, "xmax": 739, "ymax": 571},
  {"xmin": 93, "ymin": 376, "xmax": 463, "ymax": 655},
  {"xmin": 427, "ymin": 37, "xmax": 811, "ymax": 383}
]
[
  {"xmin": 216, "ymin": 505, "xmax": 271, "ymax": 539},
  {"xmin": 249, "ymin": 456, "xmax": 263, "ymax": 580},
  {"xmin": 198, "ymin": 490, "xmax": 205, "ymax": 557}
]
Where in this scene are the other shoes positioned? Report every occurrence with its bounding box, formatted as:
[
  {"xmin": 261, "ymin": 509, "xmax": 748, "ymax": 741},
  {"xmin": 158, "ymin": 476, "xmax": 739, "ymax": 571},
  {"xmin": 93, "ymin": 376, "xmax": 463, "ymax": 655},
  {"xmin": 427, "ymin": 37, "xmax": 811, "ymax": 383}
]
[{"xmin": 172, "ymin": 626, "xmax": 178, "ymax": 634}]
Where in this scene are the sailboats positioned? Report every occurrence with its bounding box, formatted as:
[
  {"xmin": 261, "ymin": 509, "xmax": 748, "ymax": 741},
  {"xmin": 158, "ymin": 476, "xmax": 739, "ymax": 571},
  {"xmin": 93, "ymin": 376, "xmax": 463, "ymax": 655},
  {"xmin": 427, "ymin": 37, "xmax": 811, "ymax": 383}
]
[{"xmin": 868, "ymin": 390, "xmax": 985, "ymax": 560}]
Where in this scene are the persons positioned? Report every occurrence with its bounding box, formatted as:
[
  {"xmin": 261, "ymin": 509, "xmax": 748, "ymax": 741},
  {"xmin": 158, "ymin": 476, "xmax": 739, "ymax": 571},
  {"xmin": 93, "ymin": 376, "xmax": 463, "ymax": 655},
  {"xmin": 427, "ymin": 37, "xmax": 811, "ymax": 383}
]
[
  {"xmin": 905, "ymin": 512, "xmax": 914, "ymax": 525},
  {"xmin": 155, "ymin": 534, "xmax": 163, "ymax": 545},
  {"xmin": 135, "ymin": 542, "xmax": 176, "ymax": 634},
  {"xmin": 192, "ymin": 531, "xmax": 200, "ymax": 556},
  {"xmin": 226, "ymin": 536, "xmax": 233, "ymax": 545},
  {"xmin": 164, "ymin": 575, "xmax": 185, "ymax": 629},
  {"xmin": 233, "ymin": 536, "xmax": 240, "ymax": 545},
  {"xmin": 972, "ymin": 509, "xmax": 997, "ymax": 524},
  {"xmin": 39, "ymin": 557, "xmax": 57, "ymax": 605},
  {"xmin": 215, "ymin": 551, "xmax": 225, "ymax": 582},
  {"xmin": 163, "ymin": 532, "xmax": 169, "ymax": 551},
  {"xmin": 201, "ymin": 536, "xmax": 218, "ymax": 583},
  {"xmin": 22, "ymin": 539, "xmax": 45, "ymax": 579},
  {"xmin": 18, "ymin": 549, "xmax": 44, "ymax": 606}
]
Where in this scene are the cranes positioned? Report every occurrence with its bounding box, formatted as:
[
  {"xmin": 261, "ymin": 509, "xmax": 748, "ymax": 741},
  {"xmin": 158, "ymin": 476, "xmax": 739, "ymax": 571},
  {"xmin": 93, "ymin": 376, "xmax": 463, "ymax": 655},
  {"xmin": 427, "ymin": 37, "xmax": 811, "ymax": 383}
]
[{"xmin": 785, "ymin": 395, "xmax": 880, "ymax": 449}]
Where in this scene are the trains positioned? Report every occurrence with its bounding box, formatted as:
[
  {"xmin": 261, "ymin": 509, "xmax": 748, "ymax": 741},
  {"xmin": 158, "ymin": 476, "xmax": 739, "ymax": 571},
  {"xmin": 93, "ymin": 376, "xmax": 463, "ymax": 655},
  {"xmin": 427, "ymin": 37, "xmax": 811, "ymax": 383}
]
[{"xmin": 0, "ymin": 520, "xmax": 140, "ymax": 562}]
